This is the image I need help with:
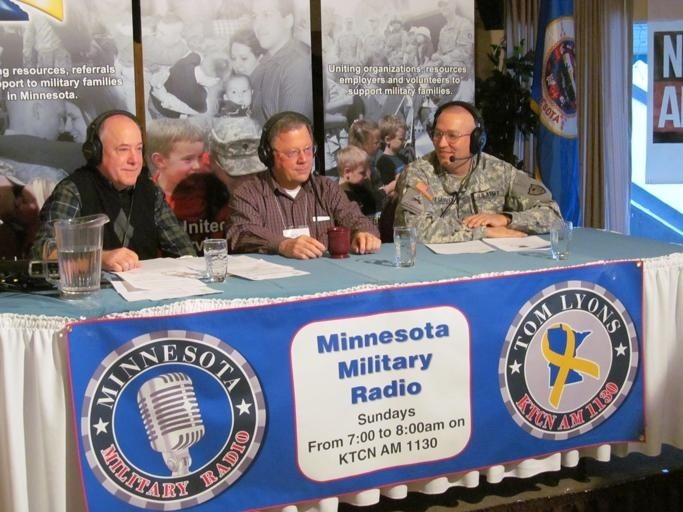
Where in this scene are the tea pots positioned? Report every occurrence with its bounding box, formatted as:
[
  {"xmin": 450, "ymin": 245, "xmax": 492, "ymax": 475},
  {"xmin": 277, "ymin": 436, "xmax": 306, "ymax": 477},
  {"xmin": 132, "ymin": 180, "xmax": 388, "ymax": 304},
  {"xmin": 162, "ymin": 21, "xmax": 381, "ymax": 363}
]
[{"xmin": 40, "ymin": 210, "xmax": 109, "ymax": 303}]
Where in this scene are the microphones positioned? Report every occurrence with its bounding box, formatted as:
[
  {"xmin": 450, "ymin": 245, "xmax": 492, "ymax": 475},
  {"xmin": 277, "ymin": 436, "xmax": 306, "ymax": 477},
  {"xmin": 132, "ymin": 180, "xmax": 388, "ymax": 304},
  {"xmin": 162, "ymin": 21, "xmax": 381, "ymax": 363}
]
[{"xmin": 449, "ymin": 155, "xmax": 479, "ymax": 162}]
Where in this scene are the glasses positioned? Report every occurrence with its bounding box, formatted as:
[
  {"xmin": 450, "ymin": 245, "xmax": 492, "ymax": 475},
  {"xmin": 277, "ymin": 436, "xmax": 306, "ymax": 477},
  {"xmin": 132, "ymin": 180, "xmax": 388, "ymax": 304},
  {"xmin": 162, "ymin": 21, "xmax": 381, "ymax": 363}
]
[
  {"xmin": 430, "ymin": 127, "xmax": 471, "ymax": 144},
  {"xmin": 271, "ymin": 143, "xmax": 313, "ymax": 157}
]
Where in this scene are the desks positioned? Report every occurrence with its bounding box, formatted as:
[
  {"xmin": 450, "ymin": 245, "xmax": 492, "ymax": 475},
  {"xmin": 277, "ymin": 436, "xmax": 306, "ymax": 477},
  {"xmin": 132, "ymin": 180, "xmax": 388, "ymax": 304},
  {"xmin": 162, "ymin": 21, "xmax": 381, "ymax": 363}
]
[{"xmin": 1, "ymin": 225, "xmax": 683, "ymax": 512}]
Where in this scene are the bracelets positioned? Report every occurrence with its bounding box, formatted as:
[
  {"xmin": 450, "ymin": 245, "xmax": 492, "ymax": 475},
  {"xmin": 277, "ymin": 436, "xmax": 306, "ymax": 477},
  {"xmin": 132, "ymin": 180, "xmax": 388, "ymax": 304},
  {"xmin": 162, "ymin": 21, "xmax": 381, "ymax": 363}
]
[{"xmin": 502, "ymin": 213, "xmax": 513, "ymax": 226}]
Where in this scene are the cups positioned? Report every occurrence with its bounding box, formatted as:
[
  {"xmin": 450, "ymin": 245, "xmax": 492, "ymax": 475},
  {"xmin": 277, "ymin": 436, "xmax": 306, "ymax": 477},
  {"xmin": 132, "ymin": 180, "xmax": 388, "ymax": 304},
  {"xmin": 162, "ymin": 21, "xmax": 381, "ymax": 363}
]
[
  {"xmin": 200, "ymin": 237, "xmax": 229, "ymax": 285},
  {"xmin": 318, "ymin": 225, "xmax": 351, "ymax": 260},
  {"xmin": 549, "ymin": 220, "xmax": 574, "ymax": 262},
  {"xmin": 392, "ymin": 226, "xmax": 420, "ymax": 267}
]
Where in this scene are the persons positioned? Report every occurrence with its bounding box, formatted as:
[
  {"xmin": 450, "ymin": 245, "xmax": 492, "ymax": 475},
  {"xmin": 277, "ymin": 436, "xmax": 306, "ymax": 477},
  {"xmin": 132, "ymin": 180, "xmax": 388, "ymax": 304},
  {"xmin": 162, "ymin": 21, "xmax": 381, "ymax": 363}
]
[
  {"xmin": 222, "ymin": 110, "xmax": 382, "ymax": 261},
  {"xmin": 145, "ymin": 2, "xmax": 313, "ymax": 235},
  {"xmin": 20, "ymin": 109, "xmax": 197, "ymax": 273},
  {"xmin": 387, "ymin": 100, "xmax": 563, "ymax": 243},
  {"xmin": 322, "ymin": 4, "xmax": 475, "ymax": 212},
  {"xmin": 1, "ymin": 1, "xmax": 129, "ymax": 217}
]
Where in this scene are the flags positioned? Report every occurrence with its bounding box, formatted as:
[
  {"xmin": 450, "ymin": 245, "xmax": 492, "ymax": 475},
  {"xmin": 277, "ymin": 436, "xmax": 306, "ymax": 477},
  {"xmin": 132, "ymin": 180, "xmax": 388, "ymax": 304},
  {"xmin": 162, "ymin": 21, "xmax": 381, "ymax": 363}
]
[{"xmin": 527, "ymin": 0, "xmax": 580, "ymax": 227}]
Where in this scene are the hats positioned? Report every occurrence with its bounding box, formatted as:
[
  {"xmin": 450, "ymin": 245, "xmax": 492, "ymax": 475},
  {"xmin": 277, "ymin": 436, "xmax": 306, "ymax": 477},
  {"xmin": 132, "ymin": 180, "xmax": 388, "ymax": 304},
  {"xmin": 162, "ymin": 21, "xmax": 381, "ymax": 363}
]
[
  {"xmin": 407, "ymin": 26, "xmax": 417, "ymax": 35},
  {"xmin": 417, "ymin": 26, "xmax": 431, "ymax": 40},
  {"xmin": 202, "ymin": 116, "xmax": 268, "ymax": 177}
]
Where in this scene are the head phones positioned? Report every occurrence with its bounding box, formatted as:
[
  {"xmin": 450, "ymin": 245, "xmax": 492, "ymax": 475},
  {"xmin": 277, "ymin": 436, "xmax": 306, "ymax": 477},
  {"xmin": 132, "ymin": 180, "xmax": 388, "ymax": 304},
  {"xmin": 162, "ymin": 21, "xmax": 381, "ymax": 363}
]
[
  {"xmin": 257, "ymin": 109, "xmax": 319, "ymax": 167},
  {"xmin": 424, "ymin": 99, "xmax": 488, "ymax": 153},
  {"xmin": 80, "ymin": 109, "xmax": 146, "ymax": 165}
]
